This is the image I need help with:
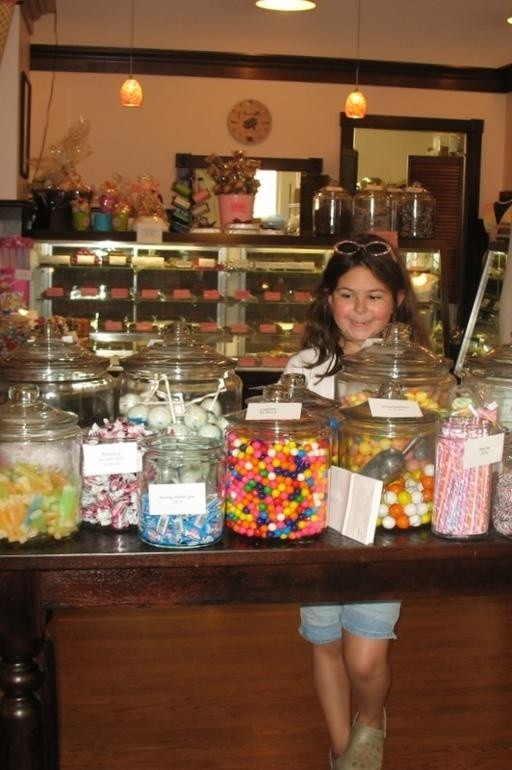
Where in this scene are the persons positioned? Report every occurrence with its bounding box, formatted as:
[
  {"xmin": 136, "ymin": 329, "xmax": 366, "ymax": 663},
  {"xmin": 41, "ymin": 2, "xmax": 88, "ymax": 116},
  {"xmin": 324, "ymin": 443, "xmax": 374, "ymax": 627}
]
[
  {"xmin": 282, "ymin": 232, "xmax": 432, "ymax": 770},
  {"xmin": 483, "ymin": 191, "xmax": 512, "ymax": 243}
]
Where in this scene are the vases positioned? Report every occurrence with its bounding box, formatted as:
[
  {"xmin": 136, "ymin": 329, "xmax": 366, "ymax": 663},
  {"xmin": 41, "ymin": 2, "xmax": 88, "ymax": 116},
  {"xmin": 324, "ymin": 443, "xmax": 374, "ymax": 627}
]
[{"xmin": 218, "ymin": 193, "xmax": 254, "ymax": 230}]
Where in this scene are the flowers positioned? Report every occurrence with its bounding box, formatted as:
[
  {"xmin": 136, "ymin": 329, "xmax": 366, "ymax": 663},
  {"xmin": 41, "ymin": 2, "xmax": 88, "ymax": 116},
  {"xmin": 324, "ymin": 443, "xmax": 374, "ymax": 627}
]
[{"xmin": 204, "ymin": 148, "xmax": 261, "ymax": 194}]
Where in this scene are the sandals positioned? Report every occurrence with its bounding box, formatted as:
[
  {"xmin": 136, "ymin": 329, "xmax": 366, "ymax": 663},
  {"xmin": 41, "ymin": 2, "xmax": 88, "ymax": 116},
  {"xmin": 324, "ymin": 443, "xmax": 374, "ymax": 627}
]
[{"xmin": 345, "ymin": 705, "xmax": 389, "ymax": 769}]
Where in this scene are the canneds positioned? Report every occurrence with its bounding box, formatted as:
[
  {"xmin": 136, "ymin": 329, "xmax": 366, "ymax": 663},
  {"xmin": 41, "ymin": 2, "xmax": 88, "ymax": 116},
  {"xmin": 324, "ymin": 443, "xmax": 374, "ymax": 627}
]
[{"xmin": 313, "ymin": 181, "xmax": 436, "ymax": 239}]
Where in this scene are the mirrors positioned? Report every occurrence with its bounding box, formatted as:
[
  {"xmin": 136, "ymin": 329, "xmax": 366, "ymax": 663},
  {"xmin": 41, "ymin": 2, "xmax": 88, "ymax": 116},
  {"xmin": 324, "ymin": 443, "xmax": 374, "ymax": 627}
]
[{"xmin": 192, "ymin": 167, "xmax": 301, "ymax": 230}]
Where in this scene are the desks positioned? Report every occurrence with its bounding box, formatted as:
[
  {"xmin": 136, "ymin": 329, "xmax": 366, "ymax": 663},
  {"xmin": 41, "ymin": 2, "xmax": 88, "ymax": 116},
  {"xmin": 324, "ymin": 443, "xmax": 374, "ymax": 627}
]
[{"xmin": 0, "ymin": 523, "xmax": 512, "ymax": 770}]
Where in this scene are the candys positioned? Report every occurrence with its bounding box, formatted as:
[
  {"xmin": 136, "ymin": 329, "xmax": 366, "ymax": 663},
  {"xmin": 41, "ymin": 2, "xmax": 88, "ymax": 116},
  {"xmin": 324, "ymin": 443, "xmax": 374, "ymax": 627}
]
[
  {"xmin": 340, "ymin": 432, "xmax": 433, "ymax": 529},
  {"xmin": 120, "ymin": 373, "xmax": 229, "ymax": 482},
  {"xmin": 333, "ymin": 387, "xmax": 447, "ymax": 468},
  {"xmin": 1, "ymin": 446, "xmax": 80, "ymax": 543},
  {"xmin": 218, "ymin": 430, "xmax": 330, "ymax": 539},
  {"xmin": 80, "ymin": 417, "xmax": 161, "ymax": 530},
  {"xmin": 432, "ymin": 397, "xmax": 497, "ymax": 537},
  {"xmin": 492, "ymin": 473, "xmax": 511, "ymax": 534},
  {"xmin": 140, "ymin": 494, "xmax": 223, "ymax": 547}
]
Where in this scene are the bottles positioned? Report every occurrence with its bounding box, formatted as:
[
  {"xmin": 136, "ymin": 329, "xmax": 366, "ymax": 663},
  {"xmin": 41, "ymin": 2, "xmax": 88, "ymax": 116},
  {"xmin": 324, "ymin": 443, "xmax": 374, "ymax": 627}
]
[
  {"xmin": 139, "ymin": 436, "xmax": 223, "ymax": 548},
  {"xmin": 114, "ymin": 323, "xmax": 244, "ymax": 438},
  {"xmin": 445, "ymin": 360, "xmax": 499, "ymax": 420},
  {"xmin": 486, "ymin": 342, "xmax": 512, "ymax": 465},
  {"xmin": 428, "ymin": 417, "xmax": 498, "ymax": 541},
  {"xmin": 1, "ymin": 384, "xmax": 84, "ymax": 547},
  {"xmin": 334, "ymin": 323, "xmax": 457, "ymax": 419},
  {"xmin": 223, "ymin": 383, "xmax": 333, "ymax": 543},
  {"xmin": 247, "ymin": 372, "xmax": 335, "ymax": 422},
  {"xmin": 490, "ymin": 432, "xmax": 512, "ymax": 537},
  {"xmin": 79, "ymin": 427, "xmax": 160, "ymax": 534},
  {"xmin": 311, "ymin": 177, "xmax": 439, "ymax": 239},
  {"xmin": 337, "ymin": 378, "xmax": 442, "ymax": 534},
  {"xmin": 170, "ymin": 182, "xmax": 211, "ymax": 234},
  {"xmin": 2, "ymin": 321, "xmax": 114, "ymax": 429}
]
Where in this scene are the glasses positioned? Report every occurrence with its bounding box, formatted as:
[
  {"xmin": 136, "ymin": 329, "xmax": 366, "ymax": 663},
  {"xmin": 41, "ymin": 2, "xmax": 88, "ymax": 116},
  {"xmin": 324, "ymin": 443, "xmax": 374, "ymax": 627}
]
[{"xmin": 332, "ymin": 236, "xmax": 397, "ymax": 262}]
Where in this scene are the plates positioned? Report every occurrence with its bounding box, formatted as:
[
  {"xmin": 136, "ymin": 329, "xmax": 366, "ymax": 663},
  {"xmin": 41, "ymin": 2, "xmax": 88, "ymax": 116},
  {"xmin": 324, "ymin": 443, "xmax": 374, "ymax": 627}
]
[{"xmin": 188, "ymin": 222, "xmax": 284, "ymax": 236}]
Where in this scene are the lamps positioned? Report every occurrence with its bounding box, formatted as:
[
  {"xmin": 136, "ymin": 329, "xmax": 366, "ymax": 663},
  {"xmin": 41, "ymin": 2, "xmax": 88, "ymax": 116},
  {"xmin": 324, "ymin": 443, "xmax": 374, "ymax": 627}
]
[
  {"xmin": 344, "ymin": 0, "xmax": 367, "ymax": 119},
  {"xmin": 120, "ymin": 0, "xmax": 143, "ymax": 107}
]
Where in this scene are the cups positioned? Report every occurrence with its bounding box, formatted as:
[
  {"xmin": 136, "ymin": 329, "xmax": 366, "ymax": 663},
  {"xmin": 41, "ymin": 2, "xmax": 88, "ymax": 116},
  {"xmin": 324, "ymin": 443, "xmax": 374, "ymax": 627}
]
[{"xmin": 71, "ymin": 213, "xmax": 129, "ymax": 232}]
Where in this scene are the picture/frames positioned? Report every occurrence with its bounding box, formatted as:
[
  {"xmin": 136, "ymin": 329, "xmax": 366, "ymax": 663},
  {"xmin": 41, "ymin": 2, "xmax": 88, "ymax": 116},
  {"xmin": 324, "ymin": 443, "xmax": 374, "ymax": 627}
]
[{"xmin": 20, "ymin": 70, "xmax": 32, "ymax": 179}]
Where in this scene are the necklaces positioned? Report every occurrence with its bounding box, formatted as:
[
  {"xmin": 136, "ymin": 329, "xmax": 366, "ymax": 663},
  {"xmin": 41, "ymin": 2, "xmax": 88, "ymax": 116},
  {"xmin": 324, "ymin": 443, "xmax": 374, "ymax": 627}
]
[{"xmin": 497, "ymin": 199, "xmax": 512, "ymax": 204}]
